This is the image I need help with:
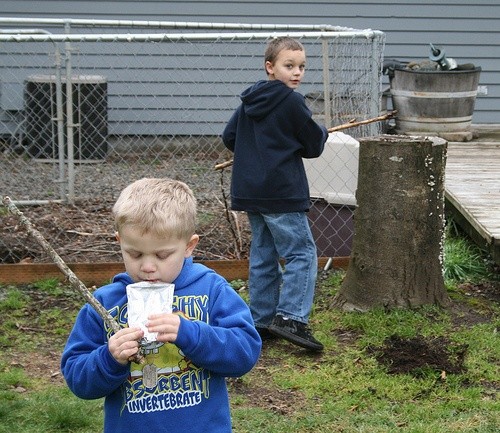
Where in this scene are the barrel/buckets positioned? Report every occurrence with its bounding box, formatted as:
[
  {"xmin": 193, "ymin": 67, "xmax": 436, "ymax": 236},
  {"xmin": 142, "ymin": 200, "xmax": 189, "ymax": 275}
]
[{"xmin": 388, "ymin": 66, "xmax": 481, "ymax": 133}]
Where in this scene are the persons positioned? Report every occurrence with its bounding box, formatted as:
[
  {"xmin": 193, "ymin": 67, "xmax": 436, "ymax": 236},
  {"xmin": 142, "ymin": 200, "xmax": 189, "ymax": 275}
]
[
  {"xmin": 59, "ymin": 177, "xmax": 262, "ymax": 433},
  {"xmin": 222, "ymin": 36, "xmax": 329, "ymax": 351}
]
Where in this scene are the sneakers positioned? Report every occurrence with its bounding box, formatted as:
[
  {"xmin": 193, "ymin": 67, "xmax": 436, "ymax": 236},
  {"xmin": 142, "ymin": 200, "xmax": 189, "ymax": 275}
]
[{"xmin": 269, "ymin": 314, "xmax": 323, "ymax": 349}]
